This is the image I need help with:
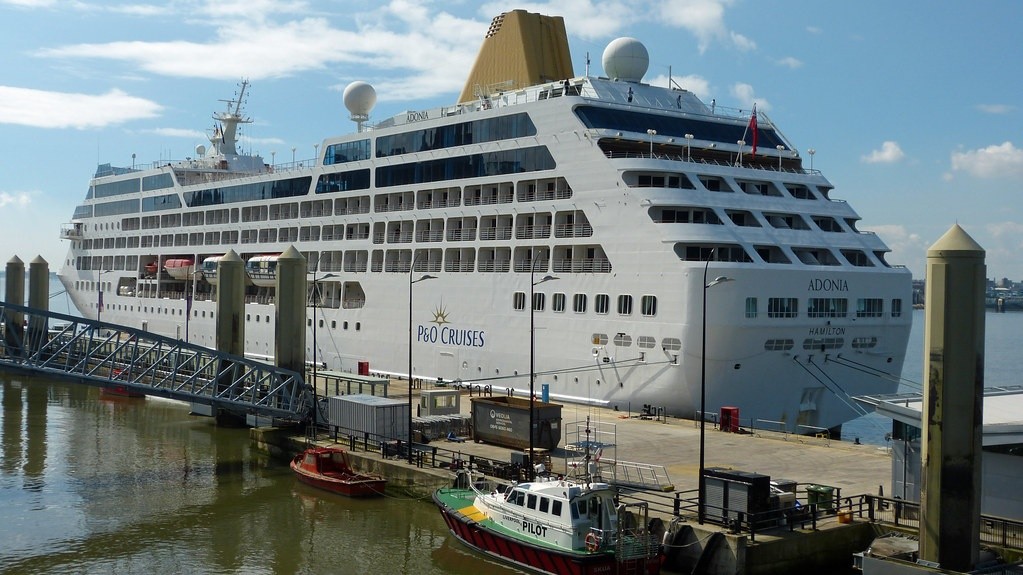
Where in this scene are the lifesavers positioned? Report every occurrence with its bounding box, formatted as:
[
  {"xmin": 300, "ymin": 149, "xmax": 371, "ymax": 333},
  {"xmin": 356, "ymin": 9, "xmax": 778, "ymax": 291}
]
[{"xmin": 585, "ymin": 532, "xmax": 599, "ymax": 552}]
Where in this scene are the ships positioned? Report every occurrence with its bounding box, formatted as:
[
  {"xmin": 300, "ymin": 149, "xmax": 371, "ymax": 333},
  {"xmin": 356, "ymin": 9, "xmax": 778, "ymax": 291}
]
[{"xmin": 53, "ymin": 9, "xmax": 914, "ymax": 435}]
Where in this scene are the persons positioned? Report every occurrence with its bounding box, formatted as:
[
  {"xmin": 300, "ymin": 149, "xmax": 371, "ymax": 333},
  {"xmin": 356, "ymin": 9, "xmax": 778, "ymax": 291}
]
[
  {"xmin": 627, "ymin": 86, "xmax": 634, "ymax": 102},
  {"xmin": 565, "ymin": 78, "xmax": 570, "ymax": 96},
  {"xmin": 677, "ymin": 94, "xmax": 682, "ymax": 109},
  {"xmin": 710, "ymin": 99, "xmax": 715, "ymax": 114}
]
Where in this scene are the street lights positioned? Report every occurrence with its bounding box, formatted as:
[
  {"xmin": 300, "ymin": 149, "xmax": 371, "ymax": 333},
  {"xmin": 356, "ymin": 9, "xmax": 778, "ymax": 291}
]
[
  {"xmin": 186, "ymin": 263, "xmax": 204, "ymax": 344},
  {"xmin": 313, "ymin": 251, "xmax": 341, "ymax": 427},
  {"xmin": 407, "ymin": 252, "xmax": 439, "ymax": 465},
  {"xmin": 697, "ymin": 246, "xmax": 729, "ymax": 526},
  {"xmin": 97, "ymin": 260, "xmax": 114, "ymax": 337},
  {"xmin": 527, "ymin": 250, "xmax": 560, "ymax": 480}
]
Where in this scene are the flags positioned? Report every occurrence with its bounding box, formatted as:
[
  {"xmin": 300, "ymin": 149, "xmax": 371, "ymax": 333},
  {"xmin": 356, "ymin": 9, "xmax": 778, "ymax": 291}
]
[{"xmin": 749, "ymin": 107, "xmax": 757, "ymax": 157}]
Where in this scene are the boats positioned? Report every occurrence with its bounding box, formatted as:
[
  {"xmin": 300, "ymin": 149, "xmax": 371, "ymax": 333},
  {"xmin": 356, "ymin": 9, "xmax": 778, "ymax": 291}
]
[
  {"xmin": 290, "ymin": 446, "xmax": 389, "ymax": 497},
  {"xmin": 246, "ymin": 255, "xmax": 277, "ymax": 287},
  {"xmin": 163, "ymin": 260, "xmax": 204, "ymax": 282},
  {"xmin": 432, "ymin": 375, "xmax": 669, "ymax": 575},
  {"xmin": 104, "ymin": 369, "xmax": 148, "ymax": 398},
  {"xmin": 203, "ymin": 256, "xmax": 224, "ymax": 286},
  {"xmin": 145, "ymin": 259, "xmax": 158, "ymax": 272}
]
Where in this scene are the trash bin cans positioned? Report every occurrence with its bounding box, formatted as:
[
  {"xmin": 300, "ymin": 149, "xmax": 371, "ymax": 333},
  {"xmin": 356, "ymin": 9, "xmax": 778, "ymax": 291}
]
[
  {"xmin": 816, "ymin": 487, "xmax": 835, "ymax": 509},
  {"xmin": 805, "ymin": 485, "xmax": 822, "ymax": 505}
]
